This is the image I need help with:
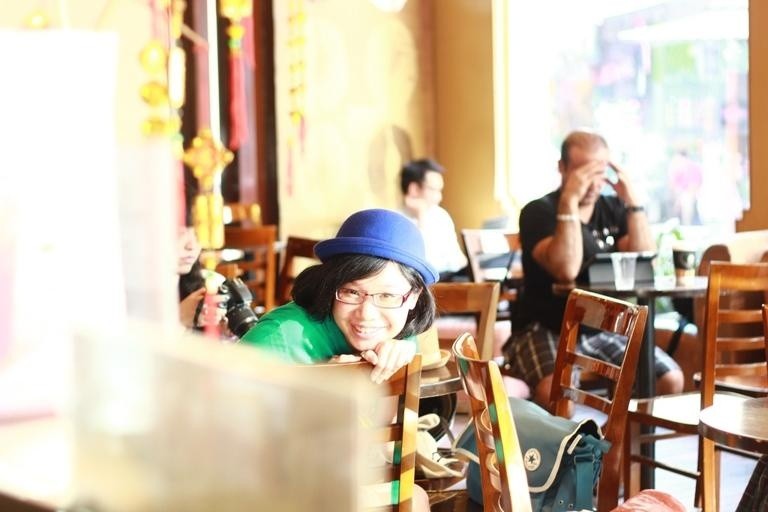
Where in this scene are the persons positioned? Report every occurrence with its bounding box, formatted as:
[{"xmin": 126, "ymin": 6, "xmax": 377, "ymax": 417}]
[
  {"xmin": 178, "ymin": 161, "xmax": 231, "ymax": 331},
  {"xmin": 396, "ymin": 158, "xmax": 470, "ymax": 284},
  {"xmin": 234, "ymin": 208, "xmax": 459, "ymax": 512},
  {"xmin": 500, "ymin": 129, "xmax": 686, "ymax": 420}
]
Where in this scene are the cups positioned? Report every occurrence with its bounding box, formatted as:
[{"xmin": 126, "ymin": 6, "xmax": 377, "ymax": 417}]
[
  {"xmin": 610, "ymin": 251, "xmax": 638, "ymax": 293},
  {"xmin": 673, "ymin": 251, "xmax": 697, "ymax": 290}
]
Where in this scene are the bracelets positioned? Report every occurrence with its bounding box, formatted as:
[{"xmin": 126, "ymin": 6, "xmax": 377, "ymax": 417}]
[
  {"xmin": 623, "ymin": 204, "xmax": 644, "ymax": 213},
  {"xmin": 555, "ymin": 213, "xmax": 582, "ymax": 222}
]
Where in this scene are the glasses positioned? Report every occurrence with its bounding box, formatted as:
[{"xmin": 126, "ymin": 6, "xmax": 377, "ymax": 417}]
[{"xmin": 334, "ymin": 287, "xmax": 415, "ymax": 307}]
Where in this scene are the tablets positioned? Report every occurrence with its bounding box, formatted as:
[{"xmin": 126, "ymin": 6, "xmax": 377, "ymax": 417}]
[{"xmin": 575, "ymin": 251, "xmax": 661, "ymax": 286}]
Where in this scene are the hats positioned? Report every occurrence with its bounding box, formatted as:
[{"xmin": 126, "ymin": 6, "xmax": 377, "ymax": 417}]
[{"xmin": 314, "ymin": 209, "xmax": 440, "ymax": 285}]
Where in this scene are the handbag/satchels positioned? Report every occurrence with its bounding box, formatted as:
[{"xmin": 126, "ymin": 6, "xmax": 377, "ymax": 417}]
[{"xmin": 452, "ymin": 395, "xmax": 612, "ymax": 511}]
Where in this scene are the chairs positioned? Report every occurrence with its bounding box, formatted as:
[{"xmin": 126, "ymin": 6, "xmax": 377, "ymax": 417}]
[
  {"xmin": 415, "ymin": 229, "xmax": 767, "ymax": 511},
  {"xmin": 221, "ymin": 225, "xmax": 320, "ymax": 313},
  {"xmin": 296, "ymin": 355, "xmax": 422, "ymax": 511}
]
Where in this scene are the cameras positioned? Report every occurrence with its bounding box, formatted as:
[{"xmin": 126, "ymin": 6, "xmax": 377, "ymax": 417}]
[{"xmin": 217, "ymin": 275, "xmax": 260, "ymax": 338}]
